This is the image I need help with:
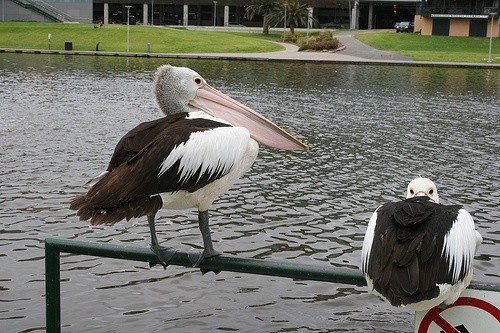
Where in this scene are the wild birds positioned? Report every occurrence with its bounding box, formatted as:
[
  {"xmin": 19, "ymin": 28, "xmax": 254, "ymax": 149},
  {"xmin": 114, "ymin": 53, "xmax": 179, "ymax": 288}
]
[
  {"xmin": 361, "ymin": 178, "xmax": 482, "ymax": 312},
  {"xmin": 70, "ymin": 64, "xmax": 308, "ymax": 276}
]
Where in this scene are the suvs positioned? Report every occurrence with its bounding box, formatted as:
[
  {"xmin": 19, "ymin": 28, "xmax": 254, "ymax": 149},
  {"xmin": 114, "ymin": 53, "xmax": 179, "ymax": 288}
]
[{"xmin": 396, "ymin": 22, "xmax": 412, "ymax": 33}]
